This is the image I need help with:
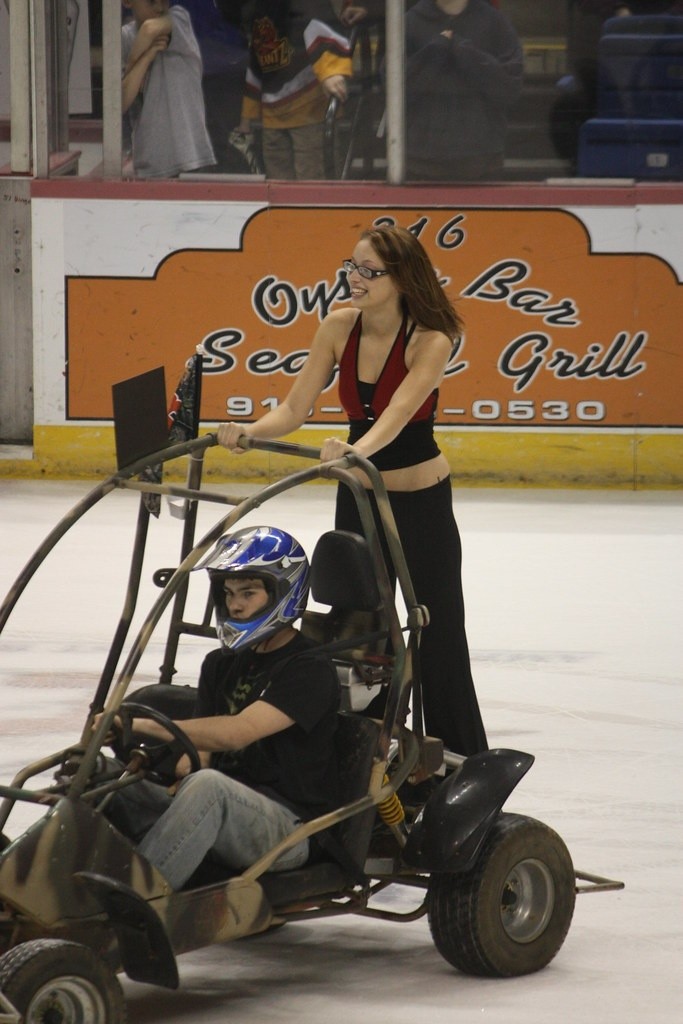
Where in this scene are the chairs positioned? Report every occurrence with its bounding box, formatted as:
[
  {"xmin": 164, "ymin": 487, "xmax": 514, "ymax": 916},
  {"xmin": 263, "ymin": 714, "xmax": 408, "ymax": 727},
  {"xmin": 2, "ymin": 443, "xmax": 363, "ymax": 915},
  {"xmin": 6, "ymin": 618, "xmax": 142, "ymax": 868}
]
[
  {"xmin": 198, "ymin": 531, "xmax": 381, "ymax": 906},
  {"xmin": 577, "ymin": 16, "xmax": 683, "ymax": 183}
]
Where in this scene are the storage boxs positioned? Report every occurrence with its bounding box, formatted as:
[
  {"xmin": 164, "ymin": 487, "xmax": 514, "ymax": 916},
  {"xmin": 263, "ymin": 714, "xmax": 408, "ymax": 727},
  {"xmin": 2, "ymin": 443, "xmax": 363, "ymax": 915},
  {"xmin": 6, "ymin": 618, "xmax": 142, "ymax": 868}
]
[{"xmin": 520, "ymin": 36, "xmax": 567, "ymax": 76}]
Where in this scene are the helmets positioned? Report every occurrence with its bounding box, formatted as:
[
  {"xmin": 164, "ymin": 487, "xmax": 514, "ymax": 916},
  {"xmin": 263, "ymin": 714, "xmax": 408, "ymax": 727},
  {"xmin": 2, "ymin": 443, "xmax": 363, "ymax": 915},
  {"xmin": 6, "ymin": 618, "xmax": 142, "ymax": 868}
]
[{"xmin": 195, "ymin": 526, "xmax": 311, "ymax": 654}]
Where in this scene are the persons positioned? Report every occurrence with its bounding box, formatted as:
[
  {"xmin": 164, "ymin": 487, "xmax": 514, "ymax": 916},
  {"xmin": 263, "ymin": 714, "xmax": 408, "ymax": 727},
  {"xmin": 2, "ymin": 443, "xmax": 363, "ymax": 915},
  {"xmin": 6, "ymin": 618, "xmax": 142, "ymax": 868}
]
[
  {"xmin": 216, "ymin": 226, "xmax": 491, "ymax": 779},
  {"xmin": 233, "ymin": 0, "xmax": 416, "ymax": 181},
  {"xmin": 122, "ymin": 0, "xmax": 218, "ymax": 179},
  {"xmin": 0, "ymin": 527, "xmax": 342, "ymax": 891},
  {"xmin": 380, "ymin": 1, "xmax": 523, "ymax": 182}
]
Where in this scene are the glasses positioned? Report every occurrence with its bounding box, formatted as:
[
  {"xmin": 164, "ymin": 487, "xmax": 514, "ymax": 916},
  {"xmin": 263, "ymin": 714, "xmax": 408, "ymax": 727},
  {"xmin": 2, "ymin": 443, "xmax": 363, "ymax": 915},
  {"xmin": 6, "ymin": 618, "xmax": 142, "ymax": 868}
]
[{"xmin": 343, "ymin": 259, "xmax": 390, "ymax": 279}]
